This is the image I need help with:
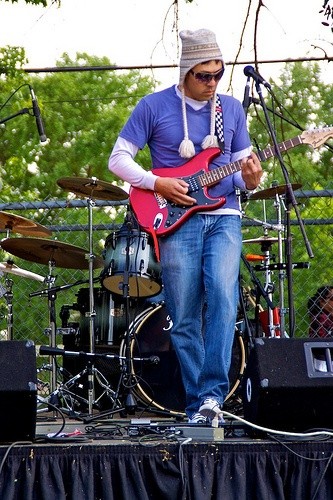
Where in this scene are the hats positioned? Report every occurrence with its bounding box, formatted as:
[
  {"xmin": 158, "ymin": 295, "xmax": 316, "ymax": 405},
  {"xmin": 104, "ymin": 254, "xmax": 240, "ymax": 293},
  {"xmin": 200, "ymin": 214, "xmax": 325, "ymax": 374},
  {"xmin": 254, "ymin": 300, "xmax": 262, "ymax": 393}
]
[{"xmin": 179, "ymin": 29, "xmax": 224, "ymax": 159}]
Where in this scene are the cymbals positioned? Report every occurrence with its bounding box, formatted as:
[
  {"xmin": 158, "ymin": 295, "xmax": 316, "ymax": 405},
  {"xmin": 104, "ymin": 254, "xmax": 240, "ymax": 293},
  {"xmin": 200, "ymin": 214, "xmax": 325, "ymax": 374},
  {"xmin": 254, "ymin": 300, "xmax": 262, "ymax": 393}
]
[
  {"xmin": 0, "ymin": 261, "xmax": 45, "ymax": 282},
  {"xmin": 57, "ymin": 177, "xmax": 130, "ymax": 201},
  {"xmin": 0, "ymin": 237, "xmax": 104, "ymax": 270},
  {"xmin": 250, "ymin": 184, "xmax": 302, "ymax": 198},
  {"xmin": 0, "ymin": 211, "xmax": 52, "ymax": 237},
  {"xmin": 241, "ymin": 236, "xmax": 284, "ymax": 244}
]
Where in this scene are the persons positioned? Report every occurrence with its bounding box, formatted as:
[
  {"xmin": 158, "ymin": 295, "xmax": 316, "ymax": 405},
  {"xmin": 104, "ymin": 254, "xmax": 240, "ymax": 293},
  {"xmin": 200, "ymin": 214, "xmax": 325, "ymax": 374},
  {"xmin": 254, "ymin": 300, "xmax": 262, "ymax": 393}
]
[
  {"xmin": 307, "ymin": 286, "xmax": 333, "ymax": 337},
  {"xmin": 108, "ymin": 30, "xmax": 263, "ymax": 424}
]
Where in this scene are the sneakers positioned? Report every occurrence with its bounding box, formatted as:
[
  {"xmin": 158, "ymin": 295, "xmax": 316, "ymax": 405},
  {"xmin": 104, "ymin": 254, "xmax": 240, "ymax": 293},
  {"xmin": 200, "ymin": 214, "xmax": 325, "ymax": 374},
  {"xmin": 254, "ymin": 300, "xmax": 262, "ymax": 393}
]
[
  {"xmin": 188, "ymin": 413, "xmax": 206, "ymax": 423},
  {"xmin": 198, "ymin": 399, "xmax": 223, "ymax": 420}
]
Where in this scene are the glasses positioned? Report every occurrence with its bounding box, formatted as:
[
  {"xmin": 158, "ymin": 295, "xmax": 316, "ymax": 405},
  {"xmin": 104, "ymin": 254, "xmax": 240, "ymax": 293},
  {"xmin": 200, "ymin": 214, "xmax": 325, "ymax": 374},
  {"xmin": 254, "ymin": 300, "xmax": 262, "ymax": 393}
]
[{"xmin": 190, "ymin": 69, "xmax": 224, "ymax": 83}]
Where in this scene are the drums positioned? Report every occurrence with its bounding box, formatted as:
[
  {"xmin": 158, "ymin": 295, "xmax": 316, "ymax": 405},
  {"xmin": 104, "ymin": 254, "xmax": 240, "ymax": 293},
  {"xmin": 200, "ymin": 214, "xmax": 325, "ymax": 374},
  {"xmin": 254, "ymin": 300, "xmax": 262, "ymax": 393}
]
[
  {"xmin": 119, "ymin": 302, "xmax": 246, "ymax": 417},
  {"xmin": 74, "ymin": 288, "xmax": 151, "ymax": 352},
  {"xmin": 99, "ymin": 229, "xmax": 164, "ymax": 300}
]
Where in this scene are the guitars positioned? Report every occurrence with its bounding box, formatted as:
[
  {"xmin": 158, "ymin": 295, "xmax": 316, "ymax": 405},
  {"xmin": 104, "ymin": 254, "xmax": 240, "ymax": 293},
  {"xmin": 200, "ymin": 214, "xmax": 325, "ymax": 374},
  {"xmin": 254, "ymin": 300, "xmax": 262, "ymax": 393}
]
[{"xmin": 129, "ymin": 124, "xmax": 333, "ymax": 238}]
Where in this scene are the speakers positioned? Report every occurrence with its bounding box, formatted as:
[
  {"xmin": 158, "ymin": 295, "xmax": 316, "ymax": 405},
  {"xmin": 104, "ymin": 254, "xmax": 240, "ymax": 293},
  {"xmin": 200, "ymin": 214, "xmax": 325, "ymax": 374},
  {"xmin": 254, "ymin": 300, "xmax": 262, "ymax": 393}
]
[
  {"xmin": 0, "ymin": 340, "xmax": 37, "ymax": 442},
  {"xmin": 241, "ymin": 337, "xmax": 333, "ymax": 437}
]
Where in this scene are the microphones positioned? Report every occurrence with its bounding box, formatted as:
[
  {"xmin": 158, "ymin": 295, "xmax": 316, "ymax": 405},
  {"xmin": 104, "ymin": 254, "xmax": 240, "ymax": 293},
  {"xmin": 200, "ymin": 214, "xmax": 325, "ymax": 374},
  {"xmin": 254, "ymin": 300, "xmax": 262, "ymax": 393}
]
[
  {"xmin": 29, "ymin": 88, "xmax": 50, "ymax": 146},
  {"xmin": 243, "ymin": 66, "xmax": 271, "ymax": 88}
]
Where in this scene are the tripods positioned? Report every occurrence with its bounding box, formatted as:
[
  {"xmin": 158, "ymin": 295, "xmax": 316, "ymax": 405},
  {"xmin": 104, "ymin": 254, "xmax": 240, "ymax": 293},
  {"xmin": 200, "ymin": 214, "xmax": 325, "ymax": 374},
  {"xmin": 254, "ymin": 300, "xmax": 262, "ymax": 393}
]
[{"xmin": 36, "ymin": 191, "xmax": 121, "ymax": 421}]
